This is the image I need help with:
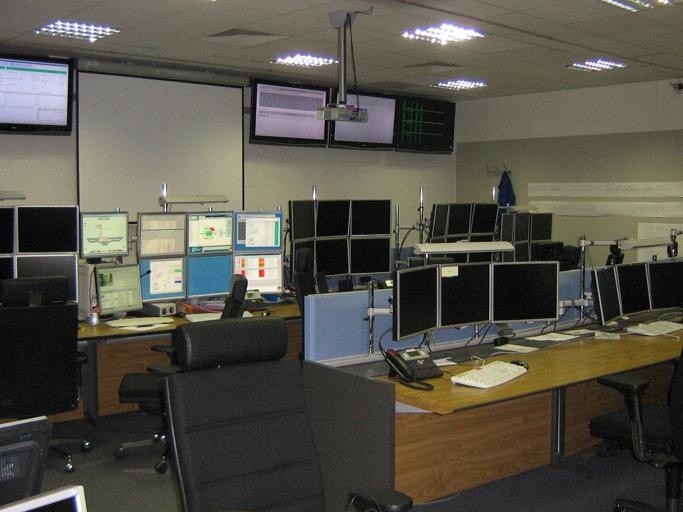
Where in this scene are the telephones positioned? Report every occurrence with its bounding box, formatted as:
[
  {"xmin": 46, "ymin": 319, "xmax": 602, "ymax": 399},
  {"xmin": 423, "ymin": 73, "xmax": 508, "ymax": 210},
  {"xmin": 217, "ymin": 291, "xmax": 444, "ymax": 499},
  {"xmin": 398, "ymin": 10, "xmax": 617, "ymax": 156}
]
[{"xmin": 386, "ymin": 346, "xmax": 444, "ymax": 382}]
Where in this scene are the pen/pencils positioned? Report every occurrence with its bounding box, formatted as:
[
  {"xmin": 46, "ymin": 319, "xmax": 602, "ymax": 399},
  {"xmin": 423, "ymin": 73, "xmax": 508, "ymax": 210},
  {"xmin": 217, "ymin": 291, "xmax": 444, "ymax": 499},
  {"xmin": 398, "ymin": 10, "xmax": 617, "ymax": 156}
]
[{"xmin": 136, "ymin": 324, "xmax": 155, "ymax": 328}]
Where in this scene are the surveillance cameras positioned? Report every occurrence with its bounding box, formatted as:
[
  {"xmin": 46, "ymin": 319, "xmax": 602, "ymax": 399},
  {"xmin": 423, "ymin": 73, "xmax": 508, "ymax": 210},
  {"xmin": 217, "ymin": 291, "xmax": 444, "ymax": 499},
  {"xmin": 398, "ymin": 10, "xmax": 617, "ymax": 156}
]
[{"xmin": 673, "ymin": 82, "xmax": 683, "ymax": 93}]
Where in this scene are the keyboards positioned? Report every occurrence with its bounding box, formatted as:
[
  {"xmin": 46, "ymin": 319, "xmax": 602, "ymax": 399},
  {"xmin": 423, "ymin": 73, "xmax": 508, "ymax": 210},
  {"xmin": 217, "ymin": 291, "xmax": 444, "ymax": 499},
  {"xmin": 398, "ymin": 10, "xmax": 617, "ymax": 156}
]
[
  {"xmin": 185, "ymin": 310, "xmax": 254, "ymax": 323},
  {"xmin": 105, "ymin": 316, "xmax": 174, "ymax": 328},
  {"xmin": 627, "ymin": 319, "xmax": 683, "ymax": 337}
]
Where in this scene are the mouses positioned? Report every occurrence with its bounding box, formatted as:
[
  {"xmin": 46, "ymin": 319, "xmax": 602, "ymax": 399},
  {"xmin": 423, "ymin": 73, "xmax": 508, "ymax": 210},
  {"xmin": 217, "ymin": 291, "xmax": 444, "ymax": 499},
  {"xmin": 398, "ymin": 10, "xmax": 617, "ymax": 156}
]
[
  {"xmin": 510, "ymin": 359, "xmax": 529, "ymax": 370},
  {"xmin": 451, "ymin": 360, "xmax": 528, "ymax": 388},
  {"xmin": 261, "ymin": 308, "xmax": 270, "ymax": 316}
]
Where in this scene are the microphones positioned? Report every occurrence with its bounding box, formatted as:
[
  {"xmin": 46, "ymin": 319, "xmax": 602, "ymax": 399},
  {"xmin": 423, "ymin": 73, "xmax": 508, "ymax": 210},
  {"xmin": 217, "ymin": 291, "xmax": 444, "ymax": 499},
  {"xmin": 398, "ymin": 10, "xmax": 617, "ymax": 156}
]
[{"xmin": 139, "ymin": 269, "xmax": 151, "ymax": 279}]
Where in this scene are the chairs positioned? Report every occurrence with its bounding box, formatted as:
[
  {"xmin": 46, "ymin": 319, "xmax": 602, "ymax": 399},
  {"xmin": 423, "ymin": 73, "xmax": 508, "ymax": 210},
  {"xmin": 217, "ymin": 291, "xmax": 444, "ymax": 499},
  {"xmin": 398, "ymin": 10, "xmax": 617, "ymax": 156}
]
[
  {"xmin": 582, "ymin": 342, "xmax": 683, "ymax": 512},
  {"xmin": 292, "ymin": 246, "xmax": 319, "ymax": 314},
  {"xmin": 0, "ymin": 301, "xmax": 93, "ymax": 474},
  {"xmin": 158, "ymin": 317, "xmax": 414, "ymax": 512},
  {"xmin": 110, "ymin": 273, "xmax": 249, "ymax": 474}
]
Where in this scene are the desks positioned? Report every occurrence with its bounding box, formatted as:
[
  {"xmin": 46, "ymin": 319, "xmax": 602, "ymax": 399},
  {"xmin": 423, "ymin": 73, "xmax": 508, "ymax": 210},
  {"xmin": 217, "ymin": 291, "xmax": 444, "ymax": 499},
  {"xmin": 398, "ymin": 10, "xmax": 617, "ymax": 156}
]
[
  {"xmin": 77, "ymin": 302, "xmax": 302, "ymax": 432},
  {"xmin": 336, "ymin": 322, "xmax": 682, "ymax": 477}
]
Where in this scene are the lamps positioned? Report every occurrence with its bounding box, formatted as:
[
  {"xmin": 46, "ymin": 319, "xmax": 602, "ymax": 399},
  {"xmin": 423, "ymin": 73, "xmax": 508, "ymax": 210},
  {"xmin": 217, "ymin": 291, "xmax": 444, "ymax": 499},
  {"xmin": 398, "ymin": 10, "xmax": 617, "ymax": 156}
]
[
  {"xmin": 412, "ymin": 240, "xmax": 516, "ymax": 266},
  {"xmin": 157, "ymin": 180, "xmax": 228, "ymax": 214},
  {"xmin": 573, "ymin": 232, "xmax": 674, "ymax": 322}
]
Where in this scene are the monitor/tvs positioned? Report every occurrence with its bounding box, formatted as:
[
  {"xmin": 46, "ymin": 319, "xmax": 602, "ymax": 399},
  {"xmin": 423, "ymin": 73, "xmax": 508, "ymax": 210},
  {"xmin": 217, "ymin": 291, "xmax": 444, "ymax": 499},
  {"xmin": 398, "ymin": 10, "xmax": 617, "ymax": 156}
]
[
  {"xmin": 0, "ymin": 52, "xmax": 75, "ymax": 137},
  {"xmin": 0, "ymin": 276, "xmax": 71, "ymax": 306},
  {"xmin": 615, "ymin": 262, "xmax": 652, "ymax": 316},
  {"xmin": 0, "ymin": 206, "xmax": 16, "ymax": 256},
  {"xmin": 137, "ymin": 257, "xmax": 187, "ymax": 303},
  {"xmin": 392, "ymin": 263, "xmax": 437, "ymax": 341},
  {"xmin": 434, "ymin": 203, "xmax": 496, "ymax": 261},
  {"xmin": 437, "ymin": 263, "xmax": 492, "ymax": 329},
  {"xmin": 393, "ymin": 95, "xmax": 457, "ymax": 155},
  {"xmin": 645, "ymin": 262, "xmax": 683, "ymax": 309},
  {"xmin": 327, "ymin": 86, "xmax": 396, "ymax": 151},
  {"xmin": 188, "ymin": 255, "xmax": 233, "ymax": 306},
  {"xmin": 0, "ymin": 258, "xmax": 14, "ymax": 276},
  {"xmin": 590, "ymin": 266, "xmax": 622, "ymax": 326},
  {"xmin": 233, "ymin": 255, "xmax": 285, "ymax": 310},
  {"xmin": 80, "ymin": 211, "xmax": 130, "ymax": 267},
  {"xmin": 14, "ymin": 255, "xmax": 79, "ymax": 303},
  {"xmin": 502, "ymin": 212, "xmax": 553, "ymax": 261},
  {"xmin": 249, "ymin": 78, "xmax": 328, "ymax": 149},
  {"xmin": 0, "ymin": 483, "xmax": 89, "ymax": 512},
  {"xmin": 136, "ymin": 211, "xmax": 188, "ymax": 258},
  {"xmin": 13, "ymin": 206, "xmax": 81, "ymax": 256},
  {"xmin": 492, "ymin": 259, "xmax": 561, "ymax": 325},
  {"xmin": 187, "ymin": 210, "xmax": 233, "ymax": 254},
  {"xmin": 233, "ymin": 211, "xmax": 285, "ymax": 255},
  {"xmin": 289, "ymin": 200, "xmax": 392, "ymax": 277},
  {"xmin": 93, "ymin": 263, "xmax": 143, "ymax": 316}
]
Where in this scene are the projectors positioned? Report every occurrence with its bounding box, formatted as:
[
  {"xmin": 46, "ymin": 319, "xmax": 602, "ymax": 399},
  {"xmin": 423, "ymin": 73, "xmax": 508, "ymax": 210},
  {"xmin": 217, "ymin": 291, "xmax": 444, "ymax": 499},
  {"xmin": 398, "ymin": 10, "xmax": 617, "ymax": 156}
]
[
  {"xmin": 145, "ymin": 303, "xmax": 179, "ymax": 315},
  {"xmin": 314, "ymin": 104, "xmax": 370, "ymax": 126}
]
